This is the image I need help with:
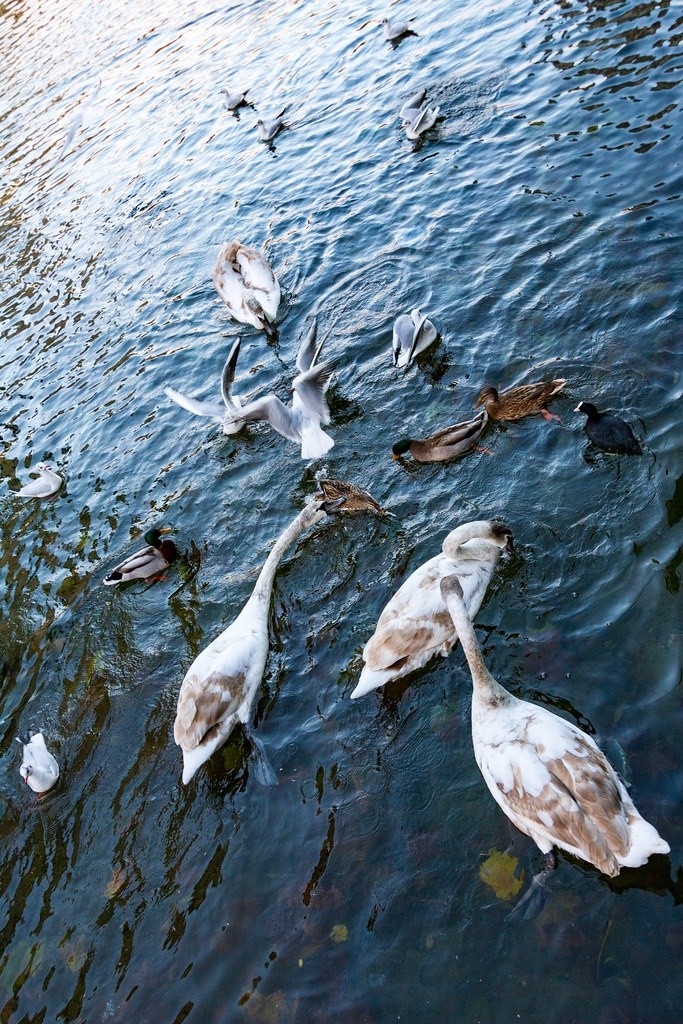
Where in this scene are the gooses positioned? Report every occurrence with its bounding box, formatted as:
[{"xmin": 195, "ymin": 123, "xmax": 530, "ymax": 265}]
[
  {"xmin": 212, "ymin": 239, "xmax": 281, "ymax": 336},
  {"xmin": 441, "ymin": 575, "xmax": 671, "ymax": 923},
  {"xmin": 173, "ymin": 497, "xmax": 347, "ymax": 785},
  {"xmin": 350, "ymin": 519, "xmax": 528, "ymax": 701}
]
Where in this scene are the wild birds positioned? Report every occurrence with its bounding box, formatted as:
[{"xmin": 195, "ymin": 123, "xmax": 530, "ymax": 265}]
[
  {"xmin": 398, "ymin": 88, "xmax": 442, "ymax": 140},
  {"xmin": 572, "ymin": 402, "xmax": 643, "ymax": 456},
  {"xmin": 253, "ymin": 109, "xmax": 285, "ymax": 141},
  {"xmin": 9, "ymin": 461, "xmax": 62, "ymax": 498},
  {"xmin": 378, "ymin": 15, "xmax": 415, "ymax": 41},
  {"xmin": 163, "ymin": 316, "xmax": 347, "ymax": 459},
  {"xmin": 392, "ymin": 307, "xmax": 437, "ymax": 368},
  {"xmin": 218, "ymin": 89, "xmax": 251, "ymax": 109},
  {"xmin": 15, "ymin": 729, "xmax": 58, "ymax": 797}
]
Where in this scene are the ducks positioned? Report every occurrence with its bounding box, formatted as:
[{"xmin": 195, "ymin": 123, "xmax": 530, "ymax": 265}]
[
  {"xmin": 313, "ymin": 479, "xmax": 389, "ymax": 518},
  {"xmin": 471, "ymin": 376, "xmax": 568, "ymax": 421},
  {"xmin": 392, "ymin": 411, "xmax": 495, "ymax": 462},
  {"xmin": 102, "ymin": 528, "xmax": 173, "ymax": 585}
]
